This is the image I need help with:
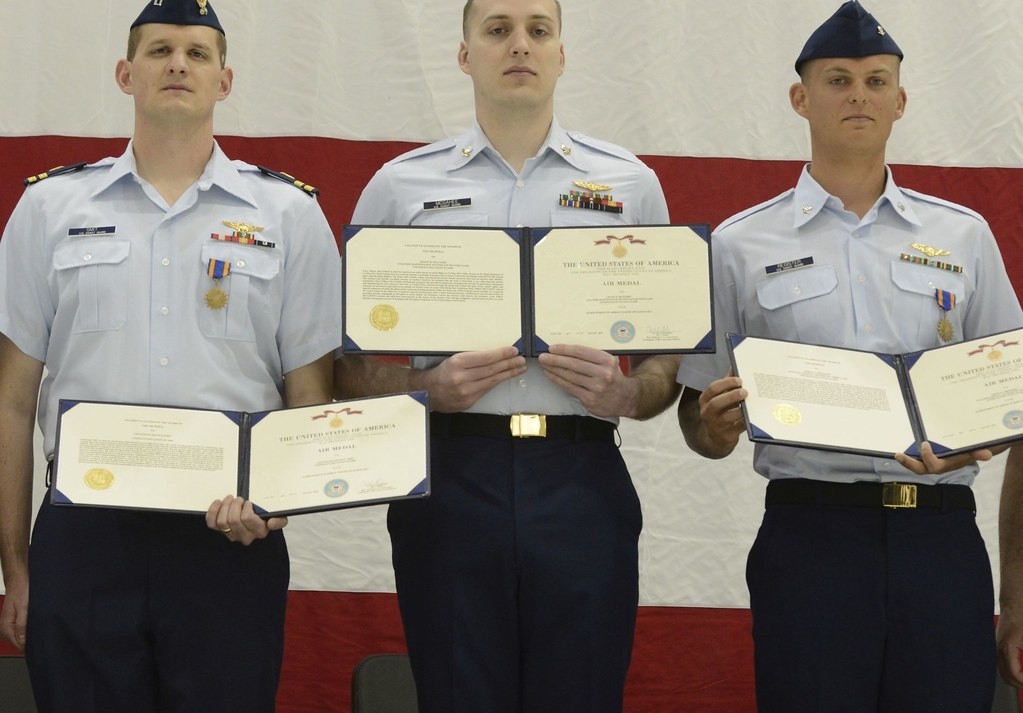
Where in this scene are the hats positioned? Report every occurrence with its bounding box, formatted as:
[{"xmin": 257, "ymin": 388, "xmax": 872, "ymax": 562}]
[
  {"xmin": 795, "ymin": 0, "xmax": 904, "ymax": 73},
  {"xmin": 129, "ymin": 1, "xmax": 224, "ymax": 34}
]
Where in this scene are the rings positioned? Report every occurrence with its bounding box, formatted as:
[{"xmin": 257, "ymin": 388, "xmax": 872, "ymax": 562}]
[{"xmin": 220, "ymin": 528, "xmax": 231, "ymax": 533}]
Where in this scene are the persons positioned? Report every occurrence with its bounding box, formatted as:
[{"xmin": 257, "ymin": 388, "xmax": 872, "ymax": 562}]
[
  {"xmin": 994, "ymin": 440, "xmax": 1021, "ymax": 691},
  {"xmin": 1, "ymin": 3, "xmax": 347, "ymax": 713},
  {"xmin": 335, "ymin": 0, "xmax": 686, "ymax": 713},
  {"xmin": 677, "ymin": 1, "xmax": 1022, "ymax": 713}
]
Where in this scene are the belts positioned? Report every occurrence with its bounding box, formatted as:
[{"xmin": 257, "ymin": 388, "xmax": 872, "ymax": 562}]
[
  {"xmin": 428, "ymin": 411, "xmax": 618, "ymax": 440},
  {"xmin": 766, "ymin": 476, "xmax": 977, "ymax": 509}
]
